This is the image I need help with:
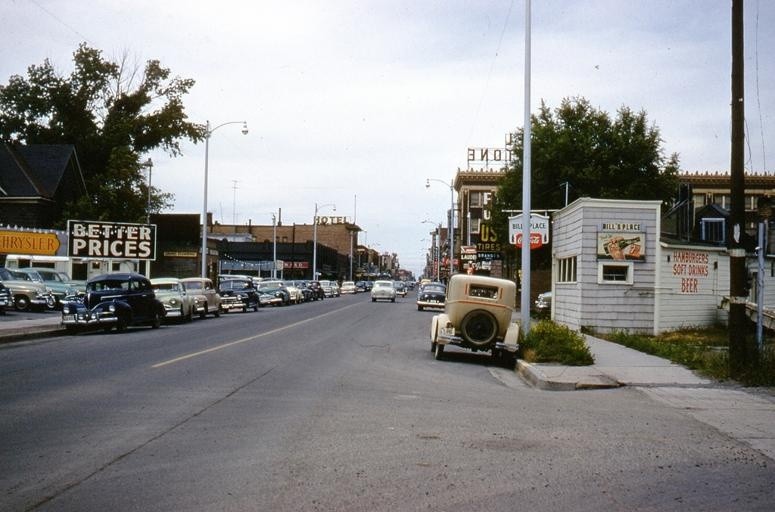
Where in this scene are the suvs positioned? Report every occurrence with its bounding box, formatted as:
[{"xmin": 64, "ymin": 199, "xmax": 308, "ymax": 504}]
[{"xmin": 428, "ymin": 273, "xmax": 525, "ymax": 368}]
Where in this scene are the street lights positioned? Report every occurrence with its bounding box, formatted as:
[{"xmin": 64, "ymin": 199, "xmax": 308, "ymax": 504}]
[
  {"xmin": 198, "ymin": 118, "xmax": 250, "ymax": 277},
  {"xmin": 137, "ymin": 158, "xmax": 157, "ymax": 277},
  {"xmin": 419, "ymin": 217, "xmax": 443, "ymax": 281},
  {"xmin": 310, "ymin": 204, "xmax": 339, "ymax": 279},
  {"xmin": 426, "ymin": 176, "xmax": 456, "ymax": 275}
]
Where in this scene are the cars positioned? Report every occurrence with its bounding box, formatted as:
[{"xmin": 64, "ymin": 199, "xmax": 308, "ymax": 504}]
[
  {"xmin": 219, "ymin": 273, "xmax": 446, "ymax": 310},
  {"xmin": 535, "ymin": 290, "xmax": 552, "ymax": 320},
  {"xmin": 1, "ymin": 250, "xmax": 218, "ymax": 334}
]
